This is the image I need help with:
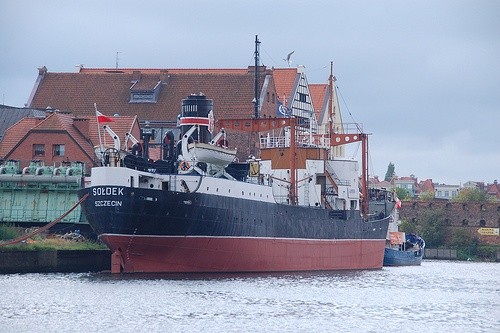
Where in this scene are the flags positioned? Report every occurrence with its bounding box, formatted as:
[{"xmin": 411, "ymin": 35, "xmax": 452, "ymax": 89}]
[
  {"xmin": 395, "ymin": 197, "xmax": 401, "ymax": 208},
  {"xmin": 96, "ymin": 111, "xmax": 116, "ymax": 124}
]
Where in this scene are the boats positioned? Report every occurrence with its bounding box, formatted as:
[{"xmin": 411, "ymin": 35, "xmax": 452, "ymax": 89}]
[
  {"xmin": 76, "ymin": 33, "xmax": 398, "ymax": 274},
  {"xmin": 383, "ymin": 232, "xmax": 426, "ymax": 267}
]
